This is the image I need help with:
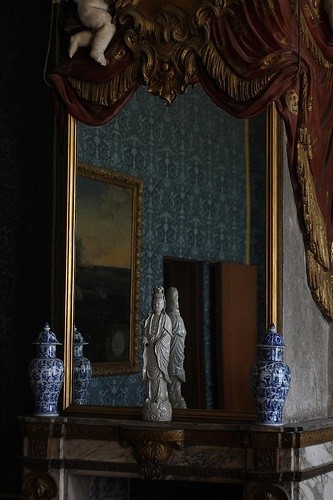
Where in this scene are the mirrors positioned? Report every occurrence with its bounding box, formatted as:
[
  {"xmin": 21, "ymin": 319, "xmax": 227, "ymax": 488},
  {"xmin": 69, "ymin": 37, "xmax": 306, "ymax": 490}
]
[{"xmin": 73, "ymin": 83, "xmax": 281, "ymax": 421}]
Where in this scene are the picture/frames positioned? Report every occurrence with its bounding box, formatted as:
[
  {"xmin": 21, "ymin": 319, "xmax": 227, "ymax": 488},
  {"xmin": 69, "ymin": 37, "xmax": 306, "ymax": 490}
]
[{"xmin": 73, "ymin": 163, "xmax": 144, "ymax": 379}]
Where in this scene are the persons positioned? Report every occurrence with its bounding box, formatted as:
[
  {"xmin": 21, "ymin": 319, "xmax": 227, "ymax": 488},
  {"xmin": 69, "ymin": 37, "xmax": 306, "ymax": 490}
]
[
  {"xmin": 142, "ymin": 284, "xmax": 173, "ymax": 403},
  {"xmin": 167, "ymin": 288, "xmax": 186, "ymax": 409}
]
